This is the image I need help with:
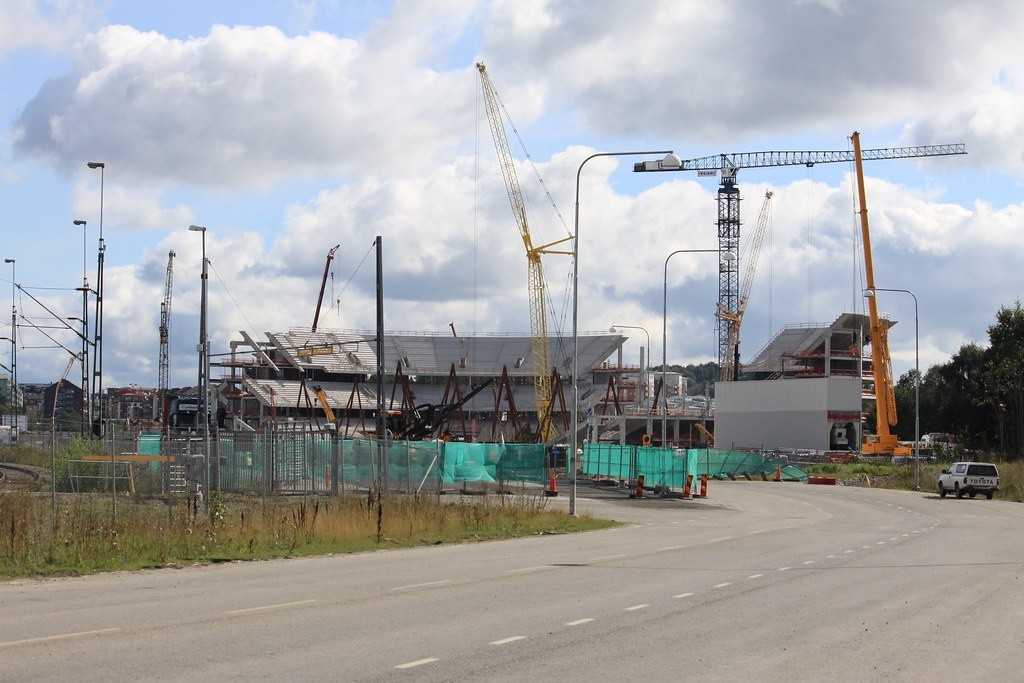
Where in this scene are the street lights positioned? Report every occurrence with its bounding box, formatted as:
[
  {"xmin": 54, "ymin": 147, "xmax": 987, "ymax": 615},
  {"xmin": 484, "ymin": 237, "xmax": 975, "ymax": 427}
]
[
  {"xmin": 863, "ymin": 288, "xmax": 923, "ymax": 492},
  {"xmin": 608, "ymin": 325, "xmax": 651, "ymax": 435},
  {"xmin": 659, "ymin": 248, "xmax": 738, "ymax": 499},
  {"xmin": 72, "ymin": 220, "xmax": 91, "ymax": 438},
  {"xmin": 4, "ymin": 258, "xmax": 18, "ymax": 447},
  {"xmin": 567, "ymin": 149, "xmax": 682, "ymax": 519},
  {"xmin": 87, "ymin": 158, "xmax": 106, "ymax": 442},
  {"xmin": 187, "ymin": 224, "xmax": 208, "ymax": 516}
]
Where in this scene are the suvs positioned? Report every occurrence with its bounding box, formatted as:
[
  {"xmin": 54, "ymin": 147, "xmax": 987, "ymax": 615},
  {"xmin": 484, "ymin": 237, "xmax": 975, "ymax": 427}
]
[{"xmin": 938, "ymin": 461, "xmax": 1000, "ymax": 499}]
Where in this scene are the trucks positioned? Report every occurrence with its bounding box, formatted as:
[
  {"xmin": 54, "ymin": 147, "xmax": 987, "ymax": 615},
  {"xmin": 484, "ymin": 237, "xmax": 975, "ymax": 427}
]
[{"xmin": 921, "ymin": 432, "xmax": 960, "ymax": 455}]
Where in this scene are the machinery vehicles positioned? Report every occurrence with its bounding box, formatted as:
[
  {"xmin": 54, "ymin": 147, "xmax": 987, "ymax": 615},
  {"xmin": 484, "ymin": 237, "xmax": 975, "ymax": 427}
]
[{"xmin": 845, "ymin": 129, "xmax": 898, "ymax": 455}]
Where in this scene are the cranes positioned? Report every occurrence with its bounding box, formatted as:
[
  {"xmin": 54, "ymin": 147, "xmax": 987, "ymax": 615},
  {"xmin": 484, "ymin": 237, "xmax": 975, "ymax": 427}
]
[
  {"xmin": 714, "ymin": 187, "xmax": 776, "ymax": 382},
  {"xmin": 632, "ymin": 141, "xmax": 968, "ymax": 382},
  {"xmin": 473, "ymin": 57, "xmax": 575, "ymax": 445},
  {"xmin": 158, "ymin": 249, "xmax": 177, "ymax": 391}
]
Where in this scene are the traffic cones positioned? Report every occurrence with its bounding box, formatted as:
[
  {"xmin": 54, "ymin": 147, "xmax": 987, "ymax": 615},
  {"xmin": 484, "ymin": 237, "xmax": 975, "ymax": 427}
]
[
  {"xmin": 545, "ymin": 470, "xmax": 558, "ymax": 497},
  {"xmin": 773, "ymin": 463, "xmax": 783, "ymax": 483}
]
